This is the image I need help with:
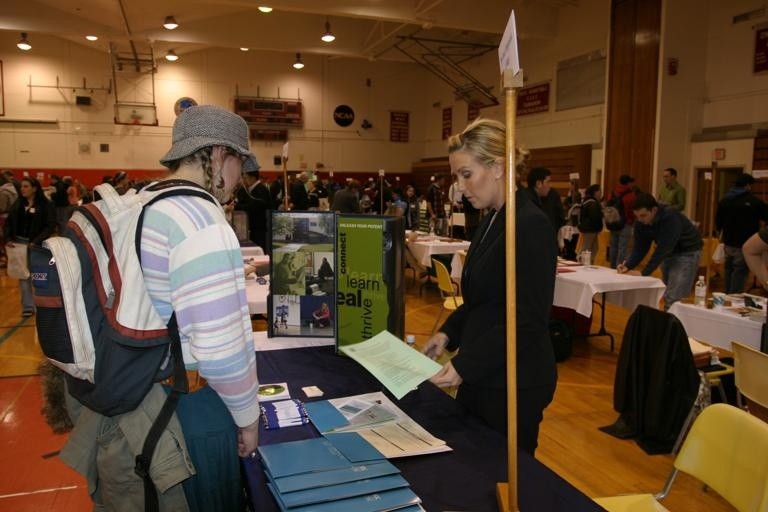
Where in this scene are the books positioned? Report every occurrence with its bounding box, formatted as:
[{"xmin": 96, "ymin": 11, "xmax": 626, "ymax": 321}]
[{"xmin": 256, "ymin": 431, "xmax": 427, "ymax": 512}]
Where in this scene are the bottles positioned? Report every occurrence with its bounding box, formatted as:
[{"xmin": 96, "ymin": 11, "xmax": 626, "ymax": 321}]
[
  {"xmin": 694, "ymin": 275, "xmax": 707, "ymax": 308},
  {"xmin": 405, "ymin": 334, "xmax": 422, "ymax": 354},
  {"xmin": 429, "ymin": 226, "xmax": 435, "ymax": 245}
]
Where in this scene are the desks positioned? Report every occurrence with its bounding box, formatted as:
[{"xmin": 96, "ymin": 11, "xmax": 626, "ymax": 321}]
[
  {"xmin": 404, "ymin": 229, "xmax": 472, "ymax": 299},
  {"xmin": 238, "ymin": 345, "xmax": 614, "ymax": 512},
  {"xmin": 243, "ymin": 253, "xmax": 270, "ymax": 319},
  {"xmin": 448, "ymin": 248, "xmax": 667, "ymax": 352},
  {"xmin": 665, "ymin": 290, "xmax": 768, "ymax": 355}
]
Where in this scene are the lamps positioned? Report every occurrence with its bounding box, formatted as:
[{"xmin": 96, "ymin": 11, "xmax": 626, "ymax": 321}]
[
  {"xmin": 319, "ymin": 22, "xmax": 336, "ymax": 44},
  {"xmin": 15, "ymin": 32, "xmax": 33, "ymax": 52},
  {"xmin": 164, "ymin": 49, "xmax": 180, "ymax": 63},
  {"xmin": 162, "ymin": 17, "xmax": 179, "ymax": 31},
  {"xmin": 291, "ymin": 52, "xmax": 305, "ymax": 71}
]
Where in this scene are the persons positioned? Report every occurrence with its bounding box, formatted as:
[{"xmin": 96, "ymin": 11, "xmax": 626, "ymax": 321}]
[
  {"xmin": 231, "ymin": 157, "xmax": 487, "ymax": 243},
  {"xmin": 271, "ymin": 251, "xmax": 298, "ymax": 295},
  {"xmin": 529, "ymin": 167, "xmax": 703, "ymax": 310},
  {"xmin": 742, "ymin": 225, "xmax": 768, "ymax": 355},
  {"xmin": 58, "ymin": 106, "xmax": 263, "ymax": 512},
  {"xmin": 423, "ymin": 118, "xmax": 558, "ymax": 459},
  {"xmin": 714, "ymin": 172, "xmax": 767, "ymax": 294},
  {"xmin": 4, "ymin": 175, "xmax": 57, "ymax": 318},
  {"xmin": 317, "ymin": 256, "xmax": 335, "ymax": 290},
  {"xmin": 0, "ymin": 172, "xmax": 152, "ymax": 268},
  {"xmin": 306, "ymin": 302, "xmax": 331, "ymax": 328}
]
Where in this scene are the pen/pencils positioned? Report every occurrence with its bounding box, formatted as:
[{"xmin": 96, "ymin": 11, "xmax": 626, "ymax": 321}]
[{"xmin": 621, "ymin": 260, "xmax": 626, "ymax": 269}]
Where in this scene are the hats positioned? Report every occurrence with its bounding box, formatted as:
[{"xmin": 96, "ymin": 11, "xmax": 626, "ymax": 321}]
[{"xmin": 159, "ymin": 106, "xmax": 261, "ymax": 174}]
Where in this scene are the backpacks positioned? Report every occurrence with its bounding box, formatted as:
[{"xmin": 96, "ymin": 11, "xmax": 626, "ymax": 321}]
[
  {"xmin": 602, "ymin": 188, "xmax": 635, "ymax": 230},
  {"xmin": 31, "ymin": 179, "xmax": 228, "ymax": 418},
  {"xmin": 565, "ymin": 199, "xmax": 598, "ymax": 226}
]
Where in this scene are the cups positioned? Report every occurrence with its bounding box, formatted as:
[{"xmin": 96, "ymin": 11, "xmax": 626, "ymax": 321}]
[
  {"xmin": 711, "ymin": 292, "xmax": 726, "ymax": 311},
  {"xmin": 582, "ymin": 249, "xmax": 592, "ymax": 266}
]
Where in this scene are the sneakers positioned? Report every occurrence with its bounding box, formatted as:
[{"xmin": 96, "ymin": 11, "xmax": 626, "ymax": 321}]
[{"xmin": 22, "ymin": 310, "xmax": 34, "ymax": 317}]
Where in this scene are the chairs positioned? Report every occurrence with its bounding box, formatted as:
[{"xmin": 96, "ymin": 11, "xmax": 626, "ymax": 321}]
[
  {"xmin": 591, "ymin": 399, "xmax": 768, "ymax": 512},
  {"xmin": 426, "ymin": 257, "xmax": 464, "ymax": 336},
  {"xmin": 728, "ymin": 337, "xmax": 768, "ymax": 411}
]
